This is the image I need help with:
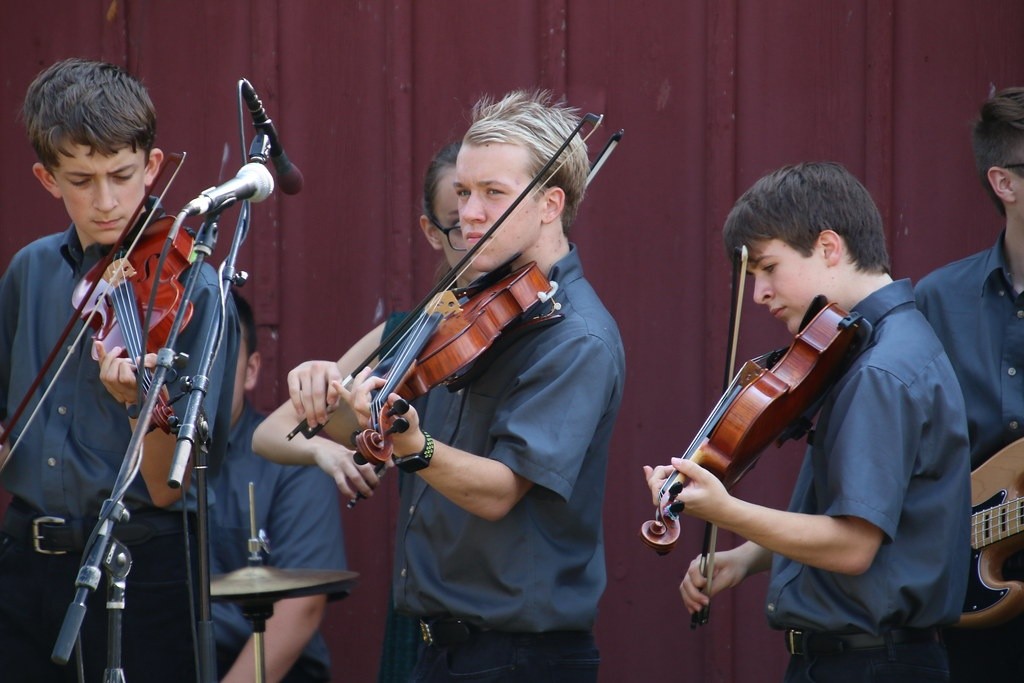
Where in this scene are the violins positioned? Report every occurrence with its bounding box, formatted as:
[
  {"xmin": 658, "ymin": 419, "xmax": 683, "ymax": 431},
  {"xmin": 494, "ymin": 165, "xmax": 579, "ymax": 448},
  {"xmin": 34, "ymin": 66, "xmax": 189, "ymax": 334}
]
[
  {"xmin": 639, "ymin": 300, "xmax": 875, "ymax": 558},
  {"xmin": 349, "ymin": 259, "xmax": 559, "ymax": 468},
  {"xmin": 70, "ymin": 215, "xmax": 197, "ymax": 437}
]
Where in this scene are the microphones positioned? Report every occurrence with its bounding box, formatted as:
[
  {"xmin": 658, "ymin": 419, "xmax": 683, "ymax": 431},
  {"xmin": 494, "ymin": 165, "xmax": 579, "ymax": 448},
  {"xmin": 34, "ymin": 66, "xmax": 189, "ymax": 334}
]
[
  {"xmin": 241, "ymin": 78, "xmax": 303, "ymax": 194},
  {"xmin": 181, "ymin": 163, "xmax": 274, "ymax": 218}
]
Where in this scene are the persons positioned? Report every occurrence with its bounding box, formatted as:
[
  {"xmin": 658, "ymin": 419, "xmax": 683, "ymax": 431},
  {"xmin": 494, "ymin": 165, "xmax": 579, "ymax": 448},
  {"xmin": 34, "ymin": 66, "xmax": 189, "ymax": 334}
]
[
  {"xmin": 208, "ymin": 287, "xmax": 351, "ymax": 683},
  {"xmin": 250, "ymin": 89, "xmax": 626, "ymax": 683},
  {"xmin": 914, "ymin": 87, "xmax": 1024, "ymax": 682},
  {"xmin": 643, "ymin": 160, "xmax": 971, "ymax": 683},
  {"xmin": 0, "ymin": 57, "xmax": 241, "ymax": 682}
]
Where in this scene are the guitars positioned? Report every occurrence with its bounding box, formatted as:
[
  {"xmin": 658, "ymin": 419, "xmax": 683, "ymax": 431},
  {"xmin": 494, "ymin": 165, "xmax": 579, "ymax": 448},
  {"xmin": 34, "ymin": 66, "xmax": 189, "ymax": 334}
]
[{"xmin": 953, "ymin": 436, "xmax": 1024, "ymax": 631}]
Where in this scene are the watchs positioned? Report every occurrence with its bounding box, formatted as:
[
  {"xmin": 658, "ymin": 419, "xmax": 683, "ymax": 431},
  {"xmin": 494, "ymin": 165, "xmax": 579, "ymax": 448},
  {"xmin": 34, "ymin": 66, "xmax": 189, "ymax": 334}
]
[{"xmin": 391, "ymin": 429, "xmax": 434, "ymax": 473}]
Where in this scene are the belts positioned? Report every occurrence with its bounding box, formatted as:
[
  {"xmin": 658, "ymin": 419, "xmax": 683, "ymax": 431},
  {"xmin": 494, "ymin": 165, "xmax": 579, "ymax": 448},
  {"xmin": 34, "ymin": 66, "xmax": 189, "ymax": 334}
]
[
  {"xmin": 405, "ymin": 609, "xmax": 451, "ymax": 648},
  {"xmin": 783, "ymin": 625, "xmax": 927, "ymax": 658},
  {"xmin": 3, "ymin": 501, "xmax": 198, "ymax": 555}
]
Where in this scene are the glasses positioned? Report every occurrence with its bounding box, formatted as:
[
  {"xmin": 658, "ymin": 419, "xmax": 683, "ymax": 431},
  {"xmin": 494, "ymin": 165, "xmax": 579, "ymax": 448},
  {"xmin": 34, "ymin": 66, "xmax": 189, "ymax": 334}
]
[{"xmin": 426, "ymin": 214, "xmax": 468, "ymax": 251}]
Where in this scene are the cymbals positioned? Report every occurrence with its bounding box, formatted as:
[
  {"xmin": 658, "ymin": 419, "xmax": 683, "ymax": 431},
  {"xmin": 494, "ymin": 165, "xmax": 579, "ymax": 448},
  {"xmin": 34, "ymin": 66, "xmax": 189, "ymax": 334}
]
[{"xmin": 209, "ymin": 563, "xmax": 360, "ymax": 606}]
